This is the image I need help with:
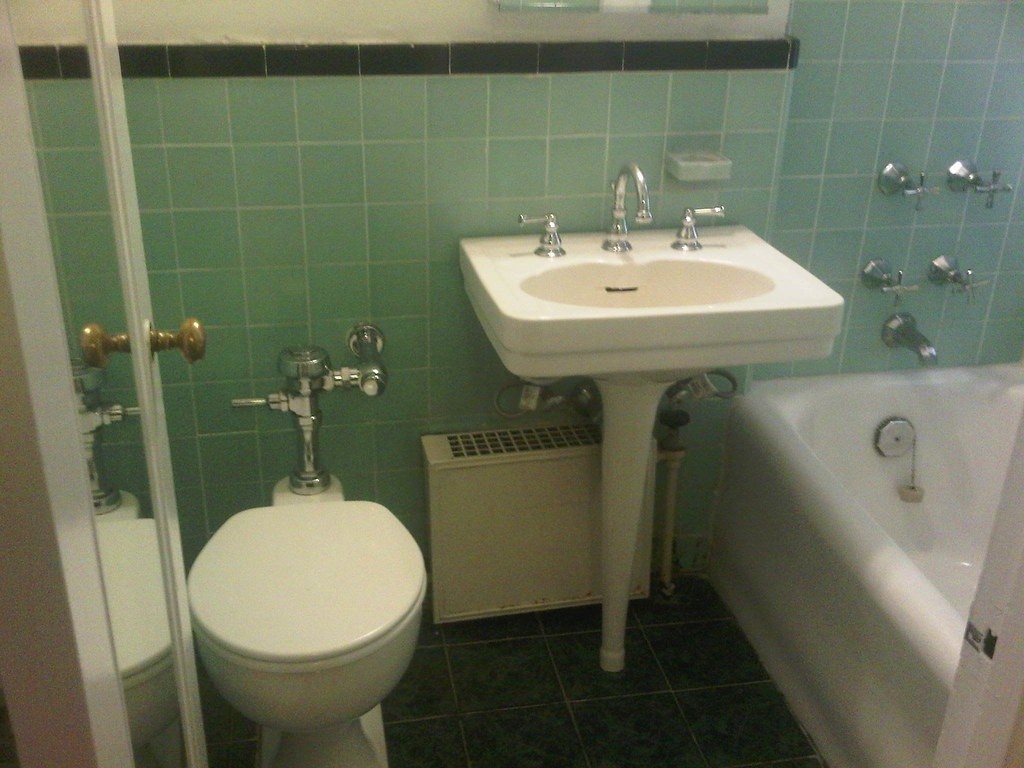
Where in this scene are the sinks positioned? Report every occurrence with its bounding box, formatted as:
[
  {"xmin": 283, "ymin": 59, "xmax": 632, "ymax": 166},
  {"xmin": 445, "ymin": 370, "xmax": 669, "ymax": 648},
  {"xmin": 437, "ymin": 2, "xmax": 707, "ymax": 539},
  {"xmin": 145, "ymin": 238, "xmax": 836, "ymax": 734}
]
[{"xmin": 461, "ymin": 227, "xmax": 846, "ymax": 379}]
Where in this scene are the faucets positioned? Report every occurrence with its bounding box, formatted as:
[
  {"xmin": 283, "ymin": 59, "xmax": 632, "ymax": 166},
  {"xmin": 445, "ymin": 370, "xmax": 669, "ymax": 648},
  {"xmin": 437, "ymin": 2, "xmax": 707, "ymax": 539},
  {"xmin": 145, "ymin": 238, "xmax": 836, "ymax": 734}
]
[
  {"xmin": 601, "ymin": 162, "xmax": 652, "ymax": 254},
  {"xmin": 883, "ymin": 313, "xmax": 938, "ymax": 368}
]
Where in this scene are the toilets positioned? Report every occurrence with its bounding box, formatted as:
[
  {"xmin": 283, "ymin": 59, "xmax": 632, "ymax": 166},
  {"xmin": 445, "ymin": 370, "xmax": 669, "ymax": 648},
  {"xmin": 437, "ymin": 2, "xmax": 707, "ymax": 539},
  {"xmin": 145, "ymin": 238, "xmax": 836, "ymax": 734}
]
[
  {"xmin": 190, "ymin": 500, "xmax": 428, "ymax": 767},
  {"xmin": 84, "ymin": 487, "xmax": 180, "ymax": 766}
]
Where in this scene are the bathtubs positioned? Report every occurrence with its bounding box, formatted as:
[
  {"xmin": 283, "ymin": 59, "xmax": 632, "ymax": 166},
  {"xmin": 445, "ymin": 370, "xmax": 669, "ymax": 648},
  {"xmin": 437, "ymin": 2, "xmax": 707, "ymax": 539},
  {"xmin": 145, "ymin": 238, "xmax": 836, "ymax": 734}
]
[{"xmin": 709, "ymin": 365, "xmax": 1018, "ymax": 767}]
